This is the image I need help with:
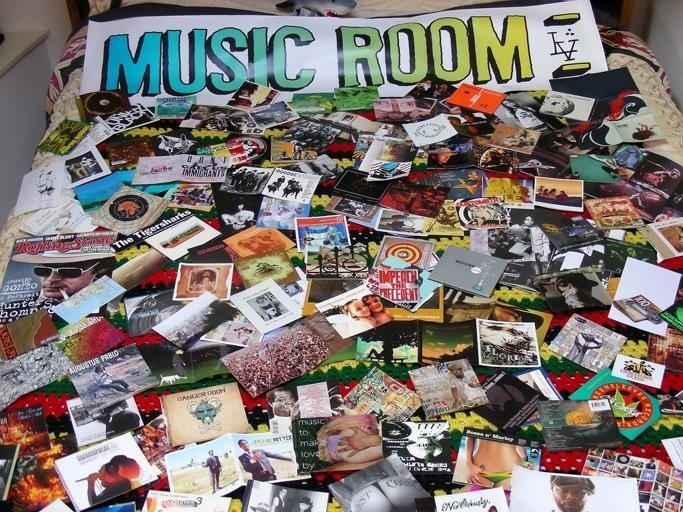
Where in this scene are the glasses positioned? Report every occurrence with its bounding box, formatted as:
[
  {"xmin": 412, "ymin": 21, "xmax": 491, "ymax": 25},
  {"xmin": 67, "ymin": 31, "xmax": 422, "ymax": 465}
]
[{"xmin": 30, "ymin": 261, "xmax": 100, "ymax": 278}]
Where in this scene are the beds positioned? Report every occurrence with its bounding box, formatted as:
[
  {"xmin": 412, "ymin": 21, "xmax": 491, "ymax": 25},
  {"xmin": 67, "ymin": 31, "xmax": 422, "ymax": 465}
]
[{"xmin": 0, "ymin": 0, "xmax": 682, "ymax": 512}]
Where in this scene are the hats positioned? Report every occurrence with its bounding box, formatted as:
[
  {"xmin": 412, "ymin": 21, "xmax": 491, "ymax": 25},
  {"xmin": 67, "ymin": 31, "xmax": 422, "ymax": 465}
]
[{"xmin": 549, "ymin": 475, "xmax": 594, "ymax": 495}]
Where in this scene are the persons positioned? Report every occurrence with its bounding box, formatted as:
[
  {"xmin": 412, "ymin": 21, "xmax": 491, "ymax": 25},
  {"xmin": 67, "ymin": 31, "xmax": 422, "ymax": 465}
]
[{"xmin": 0, "ymin": 80, "xmax": 683, "ymax": 512}]
